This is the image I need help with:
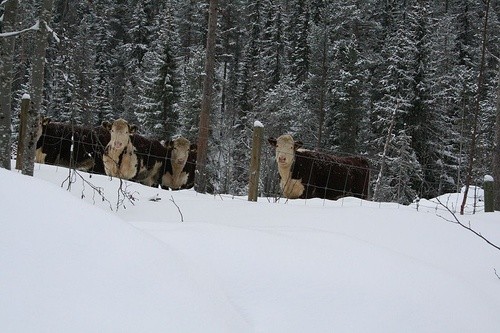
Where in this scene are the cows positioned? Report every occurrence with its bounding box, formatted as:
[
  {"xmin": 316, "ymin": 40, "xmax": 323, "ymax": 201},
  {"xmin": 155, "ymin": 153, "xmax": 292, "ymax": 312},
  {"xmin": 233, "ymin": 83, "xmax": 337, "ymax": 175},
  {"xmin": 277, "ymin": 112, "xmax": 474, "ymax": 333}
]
[
  {"xmin": 35, "ymin": 117, "xmax": 111, "ymax": 175},
  {"xmin": 162, "ymin": 136, "xmax": 196, "ymax": 190},
  {"xmin": 267, "ymin": 135, "xmax": 369, "ymax": 204},
  {"xmin": 102, "ymin": 118, "xmax": 168, "ymax": 188}
]
